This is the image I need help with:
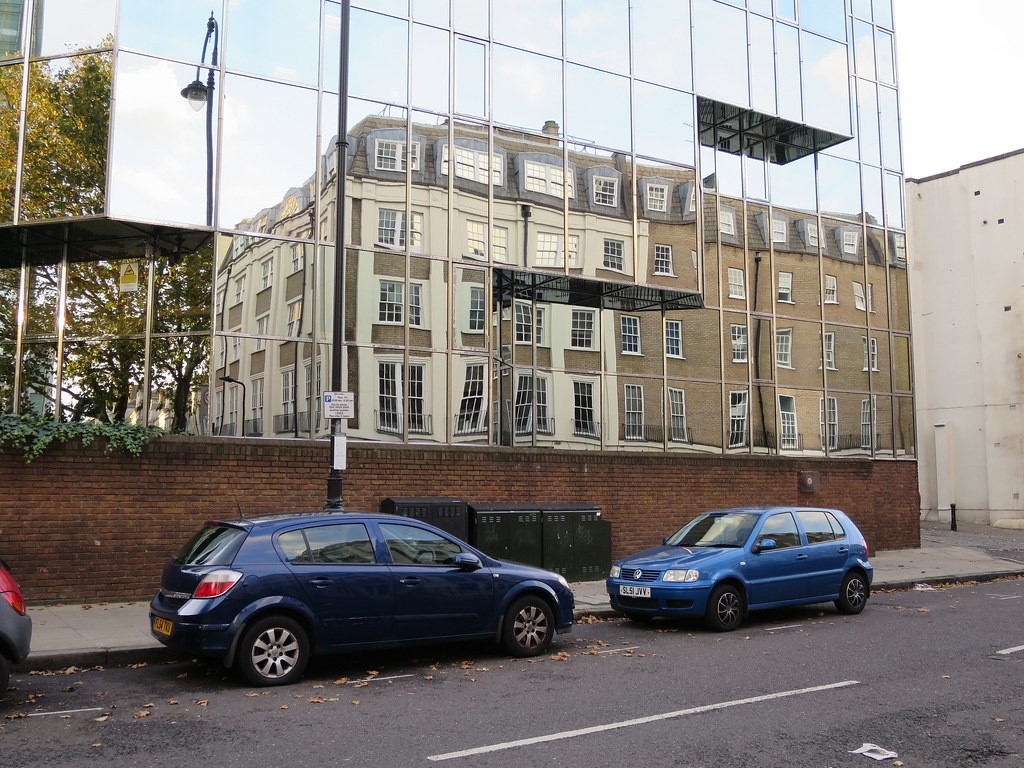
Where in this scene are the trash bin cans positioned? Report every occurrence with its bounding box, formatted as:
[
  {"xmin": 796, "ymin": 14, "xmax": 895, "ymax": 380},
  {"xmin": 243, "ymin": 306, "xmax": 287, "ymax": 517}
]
[
  {"xmin": 380, "ymin": 497, "xmax": 469, "ymax": 545},
  {"xmin": 469, "ymin": 503, "xmax": 612, "ymax": 583}
]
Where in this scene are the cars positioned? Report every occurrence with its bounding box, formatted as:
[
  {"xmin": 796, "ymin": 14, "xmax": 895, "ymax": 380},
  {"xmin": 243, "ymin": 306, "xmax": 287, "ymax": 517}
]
[
  {"xmin": 148, "ymin": 511, "xmax": 575, "ymax": 687},
  {"xmin": 605, "ymin": 506, "xmax": 875, "ymax": 634},
  {"xmin": 1, "ymin": 562, "xmax": 33, "ymax": 696}
]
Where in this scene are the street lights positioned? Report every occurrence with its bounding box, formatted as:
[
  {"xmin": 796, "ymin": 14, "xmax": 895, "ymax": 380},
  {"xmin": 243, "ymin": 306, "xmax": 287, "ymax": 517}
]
[{"xmin": 180, "ymin": 15, "xmax": 219, "ymax": 226}]
